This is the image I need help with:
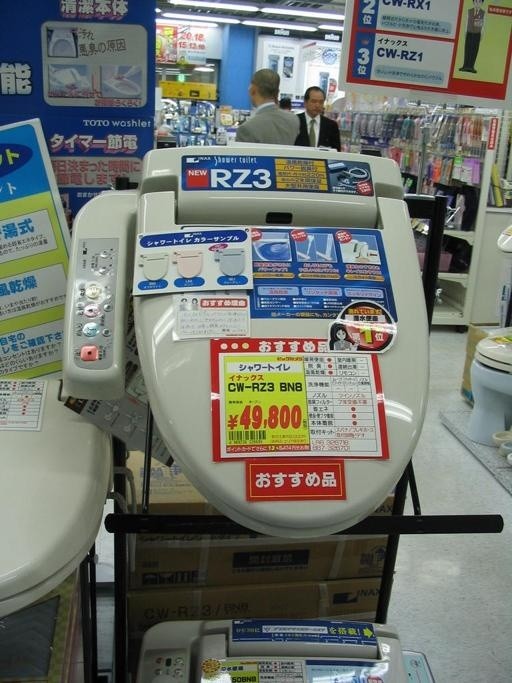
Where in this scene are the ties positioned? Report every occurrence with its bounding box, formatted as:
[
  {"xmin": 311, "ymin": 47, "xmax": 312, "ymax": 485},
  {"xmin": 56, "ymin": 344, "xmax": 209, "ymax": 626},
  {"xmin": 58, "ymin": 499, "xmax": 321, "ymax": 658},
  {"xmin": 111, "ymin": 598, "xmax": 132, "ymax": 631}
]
[{"xmin": 309, "ymin": 120, "xmax": 318, "ymax": 148}]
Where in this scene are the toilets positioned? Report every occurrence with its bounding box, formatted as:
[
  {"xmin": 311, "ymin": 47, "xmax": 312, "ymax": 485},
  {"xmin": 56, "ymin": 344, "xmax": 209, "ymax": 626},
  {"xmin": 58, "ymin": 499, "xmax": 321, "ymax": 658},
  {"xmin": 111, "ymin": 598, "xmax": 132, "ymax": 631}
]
[
  {"xmin": 137, "ymin": 616, "xmax": 433, "ymax": 683},
  {"xmin": 466, "ymin": 336, "xmax": 512, "ymax": 447},
  {"xmin": 61, "ymin": 143, "xmax": 431, "ymax": 540},
  {"xmin": 1, "ymin": 372, "xmax": 111, "ymax": 619}
]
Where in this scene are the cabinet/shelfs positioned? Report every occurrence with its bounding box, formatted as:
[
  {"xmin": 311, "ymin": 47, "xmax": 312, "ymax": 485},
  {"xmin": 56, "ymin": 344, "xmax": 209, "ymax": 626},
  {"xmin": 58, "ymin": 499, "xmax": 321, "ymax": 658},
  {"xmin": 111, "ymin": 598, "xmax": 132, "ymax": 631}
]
[{"xmin": 436, "ymin": 228, "xmax": 475, "ymax": 310}]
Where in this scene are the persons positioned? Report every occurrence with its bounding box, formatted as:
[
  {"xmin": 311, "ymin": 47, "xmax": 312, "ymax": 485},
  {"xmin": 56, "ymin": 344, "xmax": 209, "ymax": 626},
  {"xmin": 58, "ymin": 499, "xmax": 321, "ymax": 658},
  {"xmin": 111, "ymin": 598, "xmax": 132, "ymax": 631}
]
[
  {"xmin": 460, "ymin": 0, "xmax": 488, "ymax": 73},
  {"xmin": 296, "ymin": 85, "xmax": 341, "ymax": 151},
  {"xmin": 236, "ymin": 67, "xmax": 303, "ymax": 146},
  {"xmin": 280, "ymin": 96, "xmax": 292, "ymax": 114}
]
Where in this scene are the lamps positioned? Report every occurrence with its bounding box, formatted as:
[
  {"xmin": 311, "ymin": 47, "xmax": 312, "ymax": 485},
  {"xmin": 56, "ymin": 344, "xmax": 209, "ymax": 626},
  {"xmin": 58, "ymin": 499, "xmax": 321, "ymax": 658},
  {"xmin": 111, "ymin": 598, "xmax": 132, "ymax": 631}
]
[{"xmin": 155, "ymin": 0, "xmax": 346, "ymax": 32}]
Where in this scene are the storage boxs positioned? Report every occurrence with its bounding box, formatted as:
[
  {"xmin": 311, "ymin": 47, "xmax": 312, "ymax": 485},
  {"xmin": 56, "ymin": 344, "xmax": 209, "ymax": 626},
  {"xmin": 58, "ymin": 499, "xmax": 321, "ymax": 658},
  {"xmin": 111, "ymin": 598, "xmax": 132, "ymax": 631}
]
[
  {"xmin": 128, "ymin": 577, "xmax": 381, "ymax": 639},
  {"xmin": 461, "ymin": 320, "xmax": 500, "ymax": 408},
  {"xmin": 128, "ymin": 533, "xmax": 387, "ymax": 584},
  {"xmin": 128, "ymin": 448, "xmax": 395, "ymax": 518}
]
